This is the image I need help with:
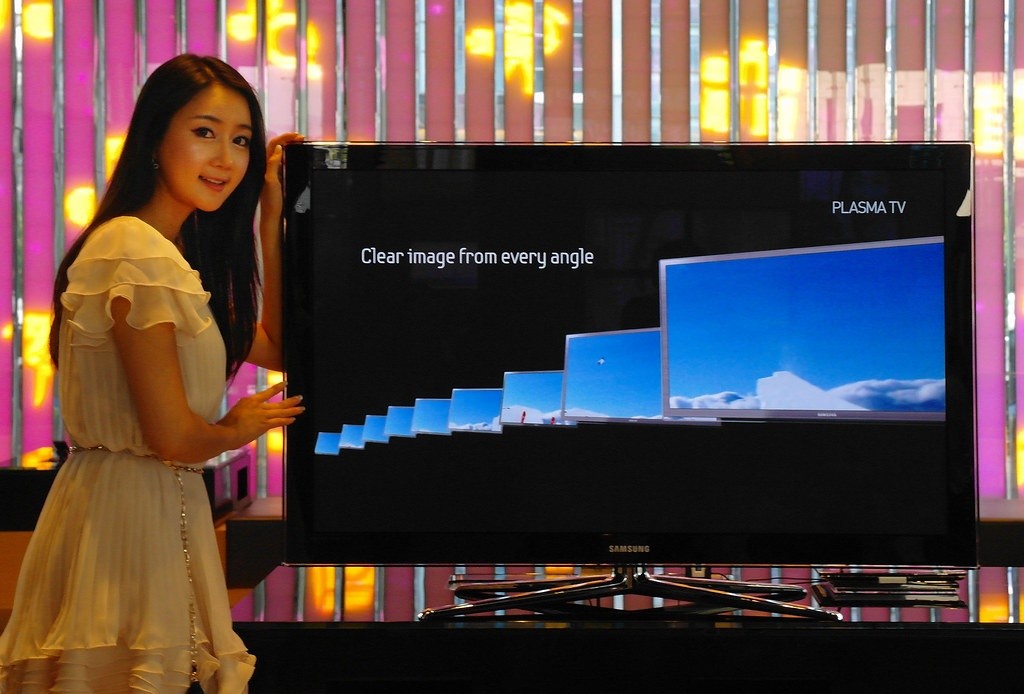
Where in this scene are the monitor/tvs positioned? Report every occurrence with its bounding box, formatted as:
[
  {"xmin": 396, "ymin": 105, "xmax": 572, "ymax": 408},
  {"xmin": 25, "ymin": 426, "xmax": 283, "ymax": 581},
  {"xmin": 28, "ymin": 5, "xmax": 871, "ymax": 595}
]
[
  {"xmin": 314, "ymin": 235, "xmax": 946, "ymax": 456},
  {"xmin": 278, "ymin": 141, "xmax": 981, "ymax": 622}
]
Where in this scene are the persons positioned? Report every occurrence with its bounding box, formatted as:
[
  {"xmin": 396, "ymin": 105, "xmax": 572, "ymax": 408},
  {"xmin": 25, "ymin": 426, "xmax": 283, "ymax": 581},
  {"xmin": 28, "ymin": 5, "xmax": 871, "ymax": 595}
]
[{"xmin": 0, "ymin": 52, "xmax": 304, "ymax": 694}]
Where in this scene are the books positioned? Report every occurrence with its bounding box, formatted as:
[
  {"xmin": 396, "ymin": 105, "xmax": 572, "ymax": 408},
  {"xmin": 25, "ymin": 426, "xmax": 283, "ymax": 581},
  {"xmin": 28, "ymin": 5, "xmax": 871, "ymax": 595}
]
[{"xmin": 810, "ymin": 573, "xmax": 966, "ymax": 606}]
